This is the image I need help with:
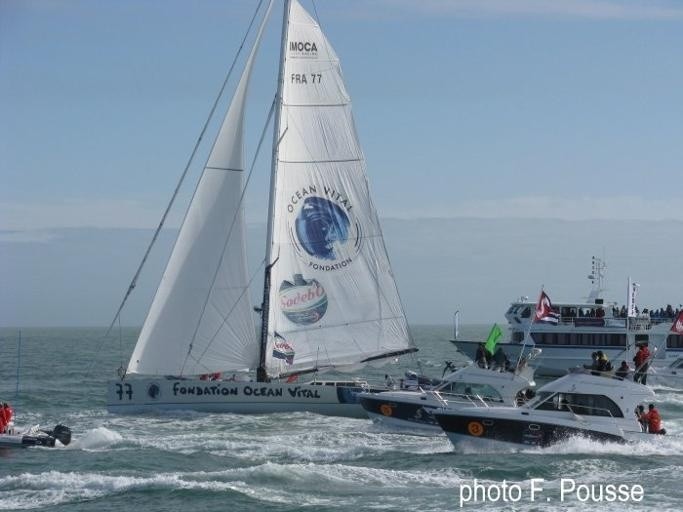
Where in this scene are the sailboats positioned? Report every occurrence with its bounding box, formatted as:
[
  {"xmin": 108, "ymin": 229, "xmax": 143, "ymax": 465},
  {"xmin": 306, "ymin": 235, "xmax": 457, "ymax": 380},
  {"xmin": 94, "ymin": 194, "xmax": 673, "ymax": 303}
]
[{"xmin": 104, "ymin": 0, "xmax": 430, "ymax": 416}]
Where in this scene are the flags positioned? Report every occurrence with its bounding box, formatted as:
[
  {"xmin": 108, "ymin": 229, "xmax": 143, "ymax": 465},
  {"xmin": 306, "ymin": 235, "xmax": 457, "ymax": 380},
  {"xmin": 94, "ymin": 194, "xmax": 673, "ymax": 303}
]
[
  {"xmin": 533, "ymin": 291, "xmax": 559, "ymax": 326},
  {"xmin": 626, "ymin": 280, "xmax": 639, "ymax": 318}
]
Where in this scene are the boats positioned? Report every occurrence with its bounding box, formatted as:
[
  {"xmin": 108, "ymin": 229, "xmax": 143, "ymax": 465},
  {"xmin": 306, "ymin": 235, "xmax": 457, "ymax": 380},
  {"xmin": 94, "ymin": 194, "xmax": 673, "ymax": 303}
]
[
  {"xmin": 353, "ymin": 348, "xmax": 544, "ymax": 439},
  {"xmin": 0, "ymin": 424, "xmax": 71, "ymax": 449},
  {"xmin": 420, "ymin": 372, "xmax": 668, "ymax": 458},
  {"xmin": 444, "ymin": 253, "xmax": 683, "ymax": 391},
  {"xmin": 564, "ymin": 339, "xmax": 682, "ymax": 395}
]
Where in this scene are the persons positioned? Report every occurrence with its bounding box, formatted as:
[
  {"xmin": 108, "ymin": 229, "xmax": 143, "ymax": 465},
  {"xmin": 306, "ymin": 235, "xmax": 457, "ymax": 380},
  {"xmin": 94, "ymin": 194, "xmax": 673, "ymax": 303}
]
[
  {"xmin": 473, "ymin": 302, "xmax": 682, "ymax": 435},
  {"xmin": 0, "ymin": 402, "xmax": 15, "ymax": 435},
  {"xmin": 198, "ymin": 370, "xmax": 300, "ymax": 383},
  {"xmin": 384, "ymin": 374, "xmax": 397, "ymax": 393}
]
